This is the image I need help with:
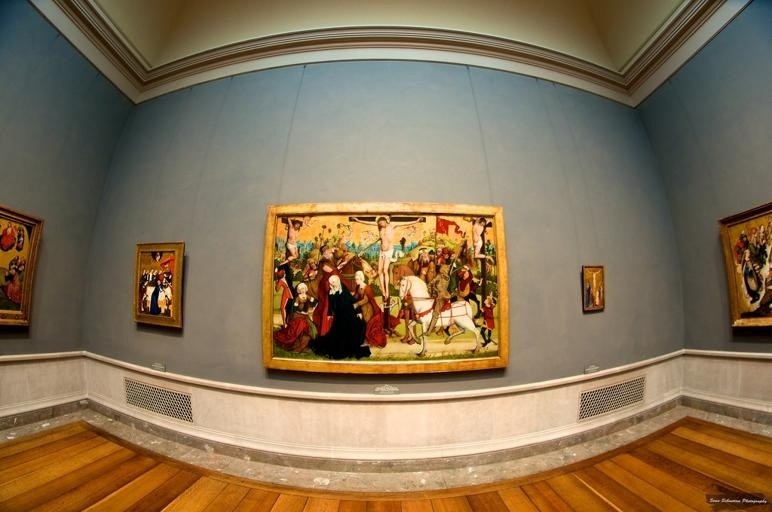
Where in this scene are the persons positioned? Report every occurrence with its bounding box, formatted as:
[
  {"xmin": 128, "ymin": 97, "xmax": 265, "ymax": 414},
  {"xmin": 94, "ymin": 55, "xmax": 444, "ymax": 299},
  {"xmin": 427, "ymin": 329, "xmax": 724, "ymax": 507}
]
[
  {"xmin": 734, "ymin": 221, "xmax": 772, "ymax": 304},
  {"xmin": 273, "ymin": 217, "xmax": 496, "ymax": 360},
  {"xmin": 139, "ymin": 269, "xmax": 172, "ymax": 317},
  {"xmin": 0, "ymin": 222, "xmax": 25, "ymax": 305},
  {"xmin": 586, "ymin": 280, "xmax": 603, "ymax": 307}
]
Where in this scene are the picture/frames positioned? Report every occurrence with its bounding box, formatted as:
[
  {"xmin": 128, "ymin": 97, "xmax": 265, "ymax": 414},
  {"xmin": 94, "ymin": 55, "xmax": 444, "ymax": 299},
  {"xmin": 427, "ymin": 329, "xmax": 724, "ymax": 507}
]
[
  {"xmin": 582, "ymin": 265, "xmax": 605, "ymax": 314},
  {"xmin": 261, "ymin": 201, "xmax": 511, "ymax": 376},
  {"xmin": 0, "ymin": 203, "xmax": 46, "ymax": 328},
  {"xmin": 715, "ymin": 200, "xmax": 772, "ymax": 328},
  {"xmin": 132, "ymin": 240, "xmax": 185, "ymax": 329}
]
